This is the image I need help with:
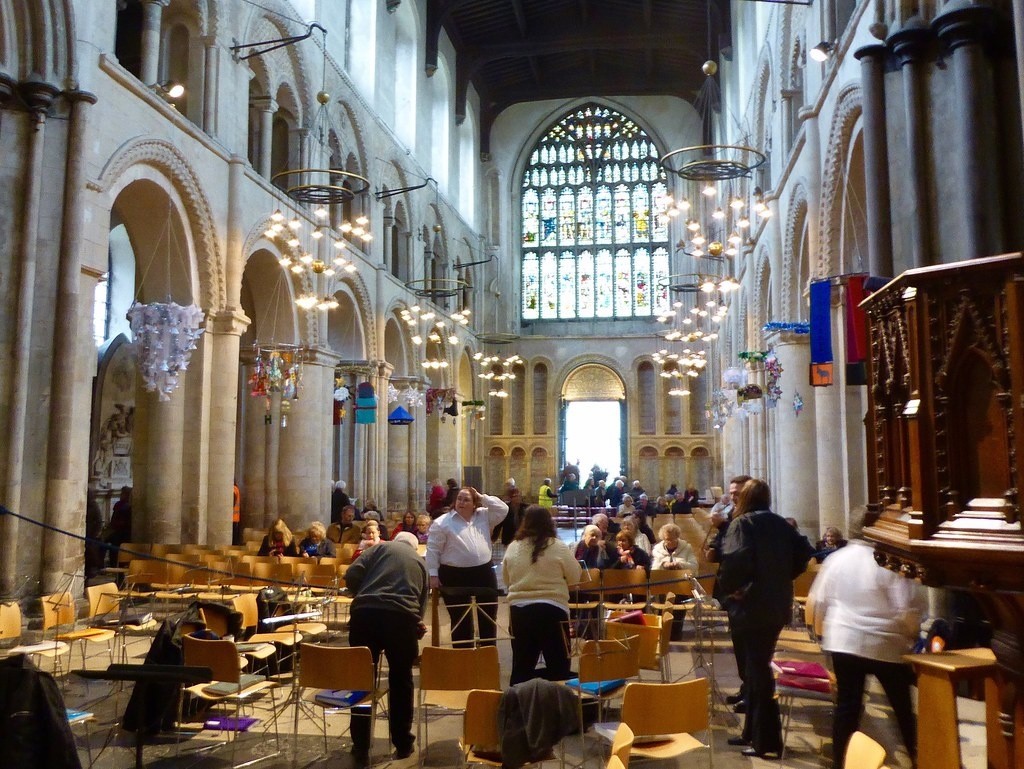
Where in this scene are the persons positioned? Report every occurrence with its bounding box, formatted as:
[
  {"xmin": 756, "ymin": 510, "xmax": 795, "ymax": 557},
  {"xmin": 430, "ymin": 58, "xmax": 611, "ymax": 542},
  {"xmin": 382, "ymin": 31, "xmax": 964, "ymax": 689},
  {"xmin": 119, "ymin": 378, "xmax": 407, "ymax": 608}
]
[
  {"xmin": 256, "ymin": 458, "xmax": 459, "ymax": 563},
  {"xmin": 706, "ymin": 474, "xmax": 849, "ymax": 758},
  {"xmin": 538, "ymin": 478, "xmax": 558, "ymax": 510},
  {"xmin": 558, "ymin": 460, "xmax": 698, "ymax": 642},
  {"xmin": 232, "ymin": 480, "xmax": 241, "ymax": 545},
  {"xmin": 426, "ymin": 487, "xmax": 509, "ymax": 648},
  {"xmin": 809, "ymin": 505, "xmax": 930, "ymax": 769},
  {"xmin": 501, "ymin": 504, "xmax": 583, "ymax": 687},
  {"xmin": 344, "ymin": 531, "xmax": 429, "ymax": 769},
  {"xmin": 491, "ymin": 477, "xmax": 531, "ymax": 547},
  {"xmin": 84, "ymin": 485, "xmax": 134, "ymax": 587}
]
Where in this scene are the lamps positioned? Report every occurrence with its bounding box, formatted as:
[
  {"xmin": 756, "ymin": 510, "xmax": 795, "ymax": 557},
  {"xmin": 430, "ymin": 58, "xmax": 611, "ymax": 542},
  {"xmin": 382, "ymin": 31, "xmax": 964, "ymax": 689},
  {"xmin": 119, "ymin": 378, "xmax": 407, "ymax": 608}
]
[{"xmin": 123, "ymin": 1, "xmax": 830, "ymax": 430}]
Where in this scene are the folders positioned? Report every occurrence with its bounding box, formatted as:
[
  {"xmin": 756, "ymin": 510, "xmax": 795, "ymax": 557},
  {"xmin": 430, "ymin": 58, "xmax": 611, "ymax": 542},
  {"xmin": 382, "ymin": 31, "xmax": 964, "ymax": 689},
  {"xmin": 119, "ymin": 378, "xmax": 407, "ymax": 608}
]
[
  {"xmin": 316, "ymin": 689, "xmax": 366, "ymax": 707},
  {"xmin": 201, "ymin": 718, "xmax": 264, "ymax": 732},
  {"xmin": 771, "ymin": 659, "xmax": 831, "ymax": 693},
  {"xmin": 565, "ymin": 677, "xmax": 626, "ymax": 696}
]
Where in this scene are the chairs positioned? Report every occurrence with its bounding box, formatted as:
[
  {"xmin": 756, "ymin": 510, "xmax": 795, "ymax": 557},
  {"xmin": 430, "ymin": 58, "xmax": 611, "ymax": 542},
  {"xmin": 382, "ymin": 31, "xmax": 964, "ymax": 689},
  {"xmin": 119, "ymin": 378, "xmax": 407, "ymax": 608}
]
[{"xmin": 1, "ymin": 485, "xmax": 835, "ymax": 769}]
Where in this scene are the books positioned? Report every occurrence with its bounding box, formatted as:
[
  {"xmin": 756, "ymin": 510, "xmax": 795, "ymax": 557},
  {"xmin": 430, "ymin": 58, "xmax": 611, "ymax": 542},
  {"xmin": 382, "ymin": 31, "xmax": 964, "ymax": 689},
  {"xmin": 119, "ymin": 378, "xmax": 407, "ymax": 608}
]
[{"xmin": 66, "ymin": 707, "xmax": 94, "ymax": 723}]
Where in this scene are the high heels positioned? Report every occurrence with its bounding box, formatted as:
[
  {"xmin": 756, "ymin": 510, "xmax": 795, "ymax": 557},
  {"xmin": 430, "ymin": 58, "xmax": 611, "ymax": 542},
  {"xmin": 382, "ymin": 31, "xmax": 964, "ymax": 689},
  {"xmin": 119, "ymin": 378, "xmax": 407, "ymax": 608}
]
[
  {"xmin": 728, "ymin": 734, "xmax": 751, "ymax": 745},
  {"xmin": 741, "ymin": 745, "xmax": 783, "ymax": 758}
]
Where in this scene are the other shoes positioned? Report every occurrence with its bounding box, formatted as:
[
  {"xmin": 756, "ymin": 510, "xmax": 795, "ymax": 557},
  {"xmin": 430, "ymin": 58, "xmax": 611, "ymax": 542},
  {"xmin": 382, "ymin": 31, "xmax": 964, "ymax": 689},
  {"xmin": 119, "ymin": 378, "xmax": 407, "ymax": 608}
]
[
  {"xmin": 733, "ymin": 700, "xmax": 746, "ymax": 713},
  {"xmin": 351, "ymin": 746, "xmax": 368, "ymax": 766},
  {"xmin": 398, "ymin": 744, "xmax": 414, "ymax": 758},
  {"xmin": 726, "ymin": 691, "xmax": 743, "ymax": 703}
]
